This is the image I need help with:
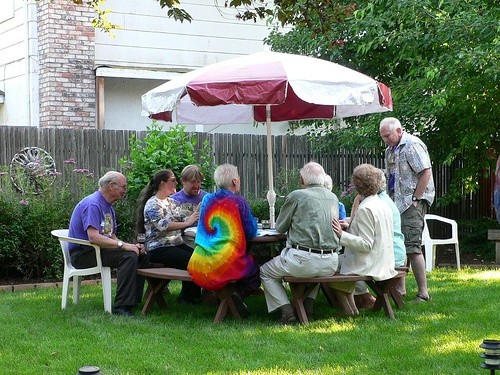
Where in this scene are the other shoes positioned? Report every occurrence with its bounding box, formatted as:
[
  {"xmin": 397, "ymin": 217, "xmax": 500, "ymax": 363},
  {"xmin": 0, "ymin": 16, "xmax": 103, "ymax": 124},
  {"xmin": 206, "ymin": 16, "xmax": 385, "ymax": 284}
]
[
  {"xmin": 280, "ymin": 313, "xmax": 296, "ymax": 323},
  {"xmin": 415, "ymin": 294, "xmax": 431, "ymax": 302}
]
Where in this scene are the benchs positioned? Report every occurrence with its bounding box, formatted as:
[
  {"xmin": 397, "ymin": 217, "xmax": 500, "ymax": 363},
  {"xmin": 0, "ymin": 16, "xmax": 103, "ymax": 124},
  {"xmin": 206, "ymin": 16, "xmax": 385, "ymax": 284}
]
[
  {"xmin": 137, "ymin": 265, "xmax": 247, "ymax": 322},
  {"xmin": 487, "ymin": 228, "xmax": 499, "ymax": 262},
  {"xmin": 283, "ymin": 267, "xmax": 410, "ymax": 326}
]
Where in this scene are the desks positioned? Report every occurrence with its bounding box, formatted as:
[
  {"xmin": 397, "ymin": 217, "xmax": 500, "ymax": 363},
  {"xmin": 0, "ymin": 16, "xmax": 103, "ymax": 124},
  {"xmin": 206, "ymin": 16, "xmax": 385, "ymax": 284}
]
[{"xmin": 183, "ymin": 229, "xmax": 287, "ymax": 247}]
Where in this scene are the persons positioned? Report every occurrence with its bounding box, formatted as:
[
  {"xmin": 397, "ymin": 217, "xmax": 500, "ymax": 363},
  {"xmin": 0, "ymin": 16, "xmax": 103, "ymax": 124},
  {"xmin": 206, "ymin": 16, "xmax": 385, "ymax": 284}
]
[
  {"xmin": 187, "ymin": 163, "xmax": 261, "ymax": 318},
  {"xmin": 339, "ymin": 168, "xmax": 408, "ymax": 309},
  {"xmin": 379, "ymin": 117, "xmax": 436, "ymax": 304},
  {"xmin": 67, "ymin": 171, "xmax": 146, "ymax": 317},
  {"xmin": 168, "ymin": 165, "xmax": 215, "ymax": 299},
  {"xmin": 135, "ymin": 169, "xmax": 205, "ymax": 306},
  {"xmin": 258, "ymin": 160, "xmax": 342, "ymax": 324},
  {"xmin": 320, "ymin": 163, "xmax": 398, "ymax": 319},
  {"xmin": 322, "ymin": 173, "xmax": 347, "ymax": 230}
]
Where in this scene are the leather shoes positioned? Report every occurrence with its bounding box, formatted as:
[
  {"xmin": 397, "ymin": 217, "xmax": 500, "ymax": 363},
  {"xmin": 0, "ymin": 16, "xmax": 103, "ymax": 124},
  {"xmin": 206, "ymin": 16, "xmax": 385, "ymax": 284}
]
[{"xmin": 113, "ymin": 306, "xmax": 131, "ymax": 315}]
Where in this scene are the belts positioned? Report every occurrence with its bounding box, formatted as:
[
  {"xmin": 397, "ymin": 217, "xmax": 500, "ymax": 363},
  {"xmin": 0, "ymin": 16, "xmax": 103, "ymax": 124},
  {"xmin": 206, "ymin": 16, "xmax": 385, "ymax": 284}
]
[{"xmin": 292, "ymin": 244, "xmax": 336, "ymax": 254}]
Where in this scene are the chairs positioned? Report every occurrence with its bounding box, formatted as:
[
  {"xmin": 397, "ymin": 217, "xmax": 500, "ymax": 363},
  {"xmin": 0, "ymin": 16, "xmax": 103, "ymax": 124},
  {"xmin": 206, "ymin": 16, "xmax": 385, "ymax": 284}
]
[
  {"xmin": 52, "ymin": 226, "xmax": 114, "ymax": 313},
  {"xmin": 421, "ymin": 214, "xmax": 463, "ymax": 274}
]
